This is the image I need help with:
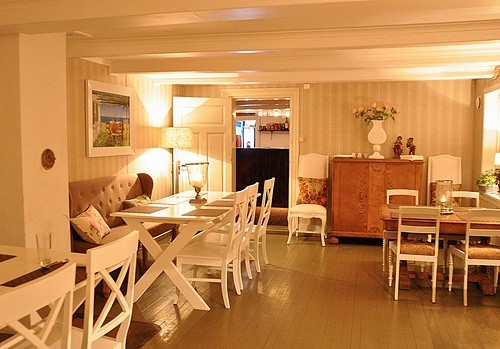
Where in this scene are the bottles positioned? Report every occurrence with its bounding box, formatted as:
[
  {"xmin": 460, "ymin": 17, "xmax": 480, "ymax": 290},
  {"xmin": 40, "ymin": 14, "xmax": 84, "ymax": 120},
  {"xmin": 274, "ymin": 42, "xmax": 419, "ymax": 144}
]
[
  {"xmin": 285, "ymin": 118, "xmax": 289, "ymax": 130},
  {"xmin": 363, "ymin": 152, "xmax": 368, "ymax": 159},
  {"xmin": 259, "ymin": 122, "xmax": 285, "ymax": 131}
]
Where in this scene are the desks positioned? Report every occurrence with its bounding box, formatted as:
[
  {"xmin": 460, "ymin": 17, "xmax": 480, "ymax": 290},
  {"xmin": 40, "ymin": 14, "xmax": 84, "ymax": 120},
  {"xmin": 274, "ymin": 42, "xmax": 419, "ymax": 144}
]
[
  {"xmin": 109, "ymin": 190, "xmax": 261, "ymax": 310},
  {"xmin": 379, "ymin": 204, "xmax": 500, "ymax": 295},
  {"xmin": 0, "ymin": 245, "xmax": 104, "ymax": 349}
]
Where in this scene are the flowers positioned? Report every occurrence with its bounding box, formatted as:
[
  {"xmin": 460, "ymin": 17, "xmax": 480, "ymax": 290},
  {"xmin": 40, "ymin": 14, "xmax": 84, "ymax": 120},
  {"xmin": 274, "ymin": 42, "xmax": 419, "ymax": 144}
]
[
  {"xmin": 475, "ymin": 170, "xmax": 498, "ymax": 186},
  {"xmin": 352, "ymin": 98, "xmax": 398, "ymax": 127}
]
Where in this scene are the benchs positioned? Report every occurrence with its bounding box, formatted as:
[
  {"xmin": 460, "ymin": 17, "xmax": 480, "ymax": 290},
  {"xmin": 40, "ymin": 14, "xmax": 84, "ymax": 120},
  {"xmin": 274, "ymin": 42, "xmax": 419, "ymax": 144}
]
[{"xmin": 68, "ymin": 172, "xmax": 180, "ymax": 298}]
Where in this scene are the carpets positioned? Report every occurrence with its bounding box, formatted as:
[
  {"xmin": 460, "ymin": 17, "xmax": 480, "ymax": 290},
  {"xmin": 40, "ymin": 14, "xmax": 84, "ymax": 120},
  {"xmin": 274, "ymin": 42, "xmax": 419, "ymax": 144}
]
[{"xmin": 72, "ymin": 312, "xmax": 162, "ymax": 349}]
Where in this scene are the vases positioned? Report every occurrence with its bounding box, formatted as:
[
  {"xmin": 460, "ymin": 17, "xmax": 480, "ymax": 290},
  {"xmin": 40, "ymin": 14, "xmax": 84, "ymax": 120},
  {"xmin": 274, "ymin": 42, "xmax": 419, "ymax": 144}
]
[
  {"xmin": 368, "ymin": 119, "xmax": 387, "ymax": 160},
  {"xmin": 479, "ymin": 185, "xmax": 498, "ymax": 193}
]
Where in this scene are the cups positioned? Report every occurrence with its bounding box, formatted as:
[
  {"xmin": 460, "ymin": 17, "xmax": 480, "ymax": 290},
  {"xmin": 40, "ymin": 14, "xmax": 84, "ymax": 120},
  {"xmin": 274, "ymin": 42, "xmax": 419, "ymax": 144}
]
[
  {"xmin": 35, "ymin": 231, "xmax": 52, "ymax": 268},
  {"xmin": 357, "ymin": 153, "xmax": 362, "ymax": 158},
  {"xmin": 352, "ymin": 152, "xmax": 356, "ymax": 158}
]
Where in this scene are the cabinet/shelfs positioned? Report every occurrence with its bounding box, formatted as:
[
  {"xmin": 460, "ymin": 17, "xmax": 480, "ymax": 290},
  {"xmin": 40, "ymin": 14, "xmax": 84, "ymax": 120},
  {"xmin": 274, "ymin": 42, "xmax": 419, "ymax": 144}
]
[{"xmin": 327, "ymin": 157, "xmax": 426, "ymax": 243}]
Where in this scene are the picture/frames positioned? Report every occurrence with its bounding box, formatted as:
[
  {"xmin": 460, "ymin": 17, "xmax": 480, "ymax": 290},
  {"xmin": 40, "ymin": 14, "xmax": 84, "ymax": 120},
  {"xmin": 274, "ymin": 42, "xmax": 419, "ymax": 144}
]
[{"xmin": 84, "ymin": 79, "xmax": 137, "ymax": 158}]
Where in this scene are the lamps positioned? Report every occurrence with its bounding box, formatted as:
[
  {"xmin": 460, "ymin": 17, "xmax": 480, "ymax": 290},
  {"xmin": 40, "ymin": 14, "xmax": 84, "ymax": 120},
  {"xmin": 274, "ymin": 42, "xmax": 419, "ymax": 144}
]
[
  {"xmin": 435, "ymin": 180, "xmax": 454, "ymax": 213},
  {"xmin": 160, "ymin": 127, "xmax": 209, "ymax": 204}
]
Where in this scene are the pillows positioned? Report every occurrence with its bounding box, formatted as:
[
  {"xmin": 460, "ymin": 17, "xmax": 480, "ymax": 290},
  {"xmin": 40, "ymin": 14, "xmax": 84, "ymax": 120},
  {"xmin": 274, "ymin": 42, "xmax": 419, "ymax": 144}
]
[
  {"xmin": 69, "ymin": 204, "xmax": 111, "ymax": 245},
  {"xmin": 295, "ymin": 177, "xmax": 329, "ymax": 208},
  {"xmin": 121, "ymin": 194, "xmax": 151, "ymax": 208},
  {"xmin": 430, "ymin": 181, "xmax": 461, "ymax": 206}
]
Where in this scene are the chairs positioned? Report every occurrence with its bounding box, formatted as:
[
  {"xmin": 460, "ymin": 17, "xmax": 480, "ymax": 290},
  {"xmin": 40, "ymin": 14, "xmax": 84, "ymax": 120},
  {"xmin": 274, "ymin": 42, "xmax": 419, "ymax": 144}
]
[
  {"xmin": 287, "ymin": 153, "xmax": 329, "ymax": 247},
  {"xmin": 427, "ymin": 155, "xmax": 461, "ymax": 206},
  {"xmin": 382, "ymin": 188, "xmax": 500, "ymax": 307},
  {"xmin": 0, "ymin": 176, "xmax": 276, "ymax": 349}
]
